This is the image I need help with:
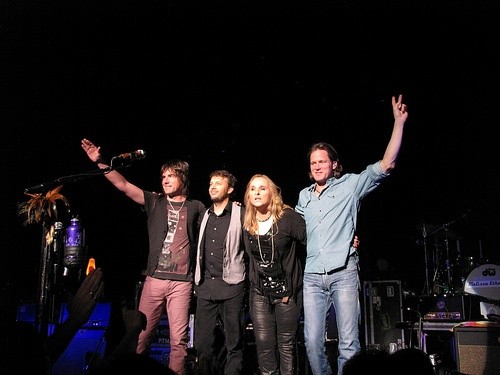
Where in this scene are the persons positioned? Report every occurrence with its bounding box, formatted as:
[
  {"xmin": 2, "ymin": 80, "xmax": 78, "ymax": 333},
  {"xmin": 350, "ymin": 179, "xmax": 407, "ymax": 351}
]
[
  {"xmin": 81, "ymin": 139, "xmax": 242, "ymax": 375},
  {"xmin": 86, "ymin": 310, "xmax": 178, "ymax": 375},
  {"xmin": 243, "ymin": 174, "xmax": 360, "ymax": 375},
  {"xmin": 342, "ymin": 348, "xmax": 436, "ymax": 375},
  {"xmin": 294, "ymin": 95, "xmax": 408, "ymax": 375},
  {"xmin": 152, "ymin": 170, "xmax": 246, "ymax": 375},
  {"xmin": 0, "ymin": 267, "xmax": 105, "ymax": 375}
]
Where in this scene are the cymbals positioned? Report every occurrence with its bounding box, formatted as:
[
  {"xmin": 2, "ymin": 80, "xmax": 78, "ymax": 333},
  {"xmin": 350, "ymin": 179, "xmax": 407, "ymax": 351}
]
[{"xmin": 418, "ymin": 224, "xmax": 464, "ymax": 241}]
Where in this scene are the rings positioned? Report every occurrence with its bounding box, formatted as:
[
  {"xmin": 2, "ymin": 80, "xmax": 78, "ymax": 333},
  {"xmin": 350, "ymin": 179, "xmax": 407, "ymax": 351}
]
[{"xmin": 88, "ymin": 289, "xmax": 94, "ymax": 297}]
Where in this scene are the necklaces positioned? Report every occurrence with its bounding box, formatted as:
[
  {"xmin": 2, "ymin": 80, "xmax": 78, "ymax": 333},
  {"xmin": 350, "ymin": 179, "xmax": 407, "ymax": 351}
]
[
  {"xmin": 254, "ymin": 213, "xmax": 280, "ymax": 267},
  {"xmin": 167, "ymin": 197, "xmax": 186, "ymax": 214}
]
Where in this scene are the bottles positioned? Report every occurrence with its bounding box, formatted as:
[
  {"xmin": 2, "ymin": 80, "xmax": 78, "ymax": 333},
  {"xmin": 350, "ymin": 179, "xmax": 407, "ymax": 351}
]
[{"xmin": 64, "ymin": 213, "xmax": 85, "ymax": 265}]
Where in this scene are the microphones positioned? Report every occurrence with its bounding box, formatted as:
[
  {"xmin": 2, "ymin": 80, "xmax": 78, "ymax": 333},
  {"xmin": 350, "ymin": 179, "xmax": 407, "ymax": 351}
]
[{"xmin": 118, "ymin": 149, "xmax": 144, "ymax": 159}]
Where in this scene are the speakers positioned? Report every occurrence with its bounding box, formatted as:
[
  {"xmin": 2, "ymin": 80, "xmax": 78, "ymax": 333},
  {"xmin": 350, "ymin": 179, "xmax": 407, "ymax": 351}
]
[
  {"xmin": 362, "ymin": 280, "xmax": 405, "ymax": 351},
  {"xmin": 454, "ymin": 327, "xmax": 500, "ymax": 374}
]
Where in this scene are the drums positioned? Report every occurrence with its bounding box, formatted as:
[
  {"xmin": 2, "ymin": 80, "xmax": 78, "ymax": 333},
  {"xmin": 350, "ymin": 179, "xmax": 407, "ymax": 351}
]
[
  {"xmin": 432, "ymin": 264, "xmax": 463, "ymax": 299},
  {"xmin": 463, "ymin": 264, "xmax": 500, "ymax": 300},
  {"xmin": 461, "ymin": 256, "xmax": 490, "ymax": 283}
]
